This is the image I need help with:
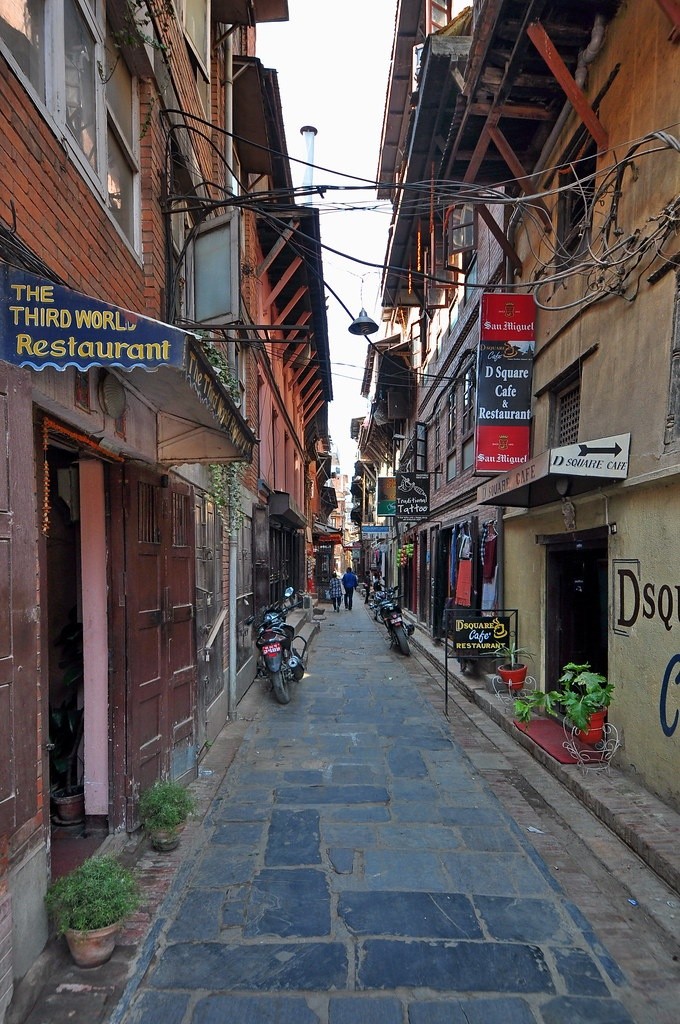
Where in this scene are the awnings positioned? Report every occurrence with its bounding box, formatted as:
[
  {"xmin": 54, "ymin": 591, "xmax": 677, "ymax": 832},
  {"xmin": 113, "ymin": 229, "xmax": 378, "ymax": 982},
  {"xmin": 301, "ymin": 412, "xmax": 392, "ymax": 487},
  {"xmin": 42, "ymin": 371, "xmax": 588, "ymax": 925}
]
[
  {"xmin": 318, "ymin": 452, "xmax": 338, "ymax": 514},
  {"xmin": 258, "ymin": 201, "xmax": 333, "ymax": 430},
  {"xmin": 0, "ymin": 258, "xmax": 261, "ymax": 466},
  {"xmin": 315, "ymin": 521, "xmax": 343, "ymax": 545},
  {"xmin": 476, "ymin": 432, "xmax": 630, "ymax": 508}
]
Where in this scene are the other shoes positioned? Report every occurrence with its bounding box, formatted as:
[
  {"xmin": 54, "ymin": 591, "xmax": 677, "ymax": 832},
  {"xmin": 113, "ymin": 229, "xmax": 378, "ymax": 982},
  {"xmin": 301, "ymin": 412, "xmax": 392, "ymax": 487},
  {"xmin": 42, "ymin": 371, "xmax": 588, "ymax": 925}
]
[
  {"xmin": 336, "ymin": 606, "xmax": 339, "ymax": 613},
  {"xmin": 349, "ymin": 608, "xmax": 352, "ymax": 610},
  {"xmin": 334, "ymin": 610, "xmax": 336, "ymax": 611},
  {"xmin": 345, "ymin": 608, "xmax": 348, "ymax": 610}
]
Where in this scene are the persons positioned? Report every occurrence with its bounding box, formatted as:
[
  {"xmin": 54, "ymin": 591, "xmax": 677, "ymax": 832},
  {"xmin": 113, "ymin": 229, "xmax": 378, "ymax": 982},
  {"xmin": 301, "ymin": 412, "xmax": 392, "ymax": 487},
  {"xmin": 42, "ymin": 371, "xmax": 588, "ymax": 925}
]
[
  {"xmin": 329, "ymin": 573, "xmax": 342, "ymax": 612},
  {"xmin": 341, "ymin": 567, "xmax": 359, "ymax": 610},
  {"xmin": 363, "ymin": 571, "xmax": 382, "ymax": 604}
]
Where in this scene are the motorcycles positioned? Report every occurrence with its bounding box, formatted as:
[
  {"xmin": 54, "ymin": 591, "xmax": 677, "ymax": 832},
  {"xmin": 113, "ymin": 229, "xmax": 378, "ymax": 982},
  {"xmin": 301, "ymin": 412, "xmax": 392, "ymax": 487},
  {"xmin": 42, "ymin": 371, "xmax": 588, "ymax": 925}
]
[
  {"xmin": 369, "ymin": 585, "xmax": 415, "ymax": 656},
  {"xmin": 243, "ymin": 586, "xmax": 308, "ymax": 704}
]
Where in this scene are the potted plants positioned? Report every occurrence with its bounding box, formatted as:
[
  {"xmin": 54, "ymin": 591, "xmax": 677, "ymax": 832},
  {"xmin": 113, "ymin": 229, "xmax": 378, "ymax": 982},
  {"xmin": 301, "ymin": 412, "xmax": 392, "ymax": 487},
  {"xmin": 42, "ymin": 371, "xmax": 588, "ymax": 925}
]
[
  {"xmin": 43, "ymin": 854, "xmax": 146, "ymax": 968},
  {"xmin": 137, "ymin": 778, "xmax": 198, "ymax": 852},
  {"xmin": 513, "ymin": 662, "xmax": 616, "ymax": 744},
  {"xmin": 479, "ymin": 641, "xmax": 535, "ymax": 690}
]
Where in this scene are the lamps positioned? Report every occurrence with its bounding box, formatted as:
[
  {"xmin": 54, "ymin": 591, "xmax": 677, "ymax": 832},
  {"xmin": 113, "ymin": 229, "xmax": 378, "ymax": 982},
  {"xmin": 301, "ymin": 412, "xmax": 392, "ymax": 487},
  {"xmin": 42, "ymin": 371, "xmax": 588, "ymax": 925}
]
[
  {"xmin": 348, "ymin": 263, "xmax": 379, "ymax": 335},
  {"xmin": 392, "ymin": 433, "xmax": 413, "ymax": 441}
]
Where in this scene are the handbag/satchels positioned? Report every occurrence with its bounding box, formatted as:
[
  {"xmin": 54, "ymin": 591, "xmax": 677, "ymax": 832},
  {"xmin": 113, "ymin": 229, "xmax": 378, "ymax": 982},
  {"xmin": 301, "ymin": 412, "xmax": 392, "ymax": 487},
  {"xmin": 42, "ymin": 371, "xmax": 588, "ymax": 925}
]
[
  {"xmin": 340, "ymin": 582, "xmax": 346, "ymax": 595},
  {"xmin": 324, "ymin": 590, "xmax": 331, "ymax": 600}
]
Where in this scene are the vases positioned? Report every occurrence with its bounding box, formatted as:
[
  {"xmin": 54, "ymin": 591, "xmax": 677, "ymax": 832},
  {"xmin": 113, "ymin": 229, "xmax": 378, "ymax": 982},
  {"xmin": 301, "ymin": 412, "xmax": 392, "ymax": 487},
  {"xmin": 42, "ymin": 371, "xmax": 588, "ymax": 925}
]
[{"xmin": 53, "ymin": 784, "xmax": 84, "ymax": 821}]
